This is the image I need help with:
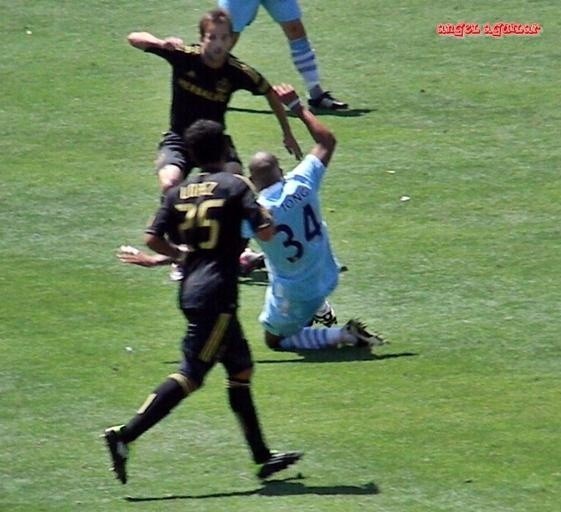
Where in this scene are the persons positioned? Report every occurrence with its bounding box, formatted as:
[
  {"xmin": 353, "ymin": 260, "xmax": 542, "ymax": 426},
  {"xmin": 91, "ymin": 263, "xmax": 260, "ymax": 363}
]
[
  {"xmin": 117, "ymin": 244, "xmax": 348, "ymax": 283},
  {"xmin": 237, "ymin": 81, "xmax": 388, "ymax": 350},
  {"xmin": 126, "ymin": 9, "xmax": 304, "ymax": 283},
  {"xmin": 103, "ymin": 118, "xmax": 306, "ymax": 484},
  {"xmin": 218, "ymin": 0, "xmax": 349, "ymax": 111}
]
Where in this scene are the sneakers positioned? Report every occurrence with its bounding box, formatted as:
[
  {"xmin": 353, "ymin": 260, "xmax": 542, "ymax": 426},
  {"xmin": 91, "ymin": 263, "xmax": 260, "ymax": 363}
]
[
  {"xmin": 253, "ymin": 450, "xmax": 305, "ymax": 479},
  {"xmin": 308, "ymin": 91, "xmax": 348, "ymax": 110},
  {"xmin": 170, "ymin": 262, "xmax": 185, "ymax": 281},
  {"xmin": 100, "ymin": 423, "xmax": 130, "ymax": 483},
  {"xmin": 341, "ymin": 319, "xmax": 385, "ymax": 346},
  {"xmin": 240, "ymin": 248, "xmax": 265, "ymax": 270},
  {"xmin": 306, "ymin": 308, "xmax": 337, "ymax": 328}
]
